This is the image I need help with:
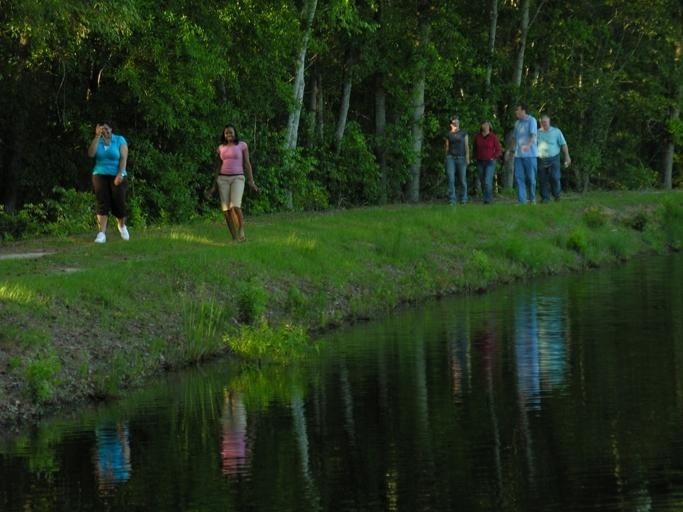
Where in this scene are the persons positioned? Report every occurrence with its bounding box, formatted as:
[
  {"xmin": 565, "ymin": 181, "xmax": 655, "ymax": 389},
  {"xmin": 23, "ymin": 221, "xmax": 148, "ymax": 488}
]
[
  {"xmin": 474, "ymin": 120, "xmax": 500, "ymax": 206},
  {"xmin": 444, "ymin": 115, "xmax": 471, "ymax": 208},
  {"xmin": 536, "ymin": 113, "xmax": 572, "ymax": 205},
  {"xmin": 216, "ymin": 124, "xmax": 259, "ymax": 242},
  {"xmin": 92, "ymin": 415, "xmax": 133, "ymax": 504},
  {"xmin": 217, "ymin": 387, "xmax": 252, "ymax": 493},
  {"xmin": 85, "ymin": 121, "xmax": 130, "ymax": 244},
  {"xmin": 504, "ymin": 104, "xmax": 539, "ymax": 206}
]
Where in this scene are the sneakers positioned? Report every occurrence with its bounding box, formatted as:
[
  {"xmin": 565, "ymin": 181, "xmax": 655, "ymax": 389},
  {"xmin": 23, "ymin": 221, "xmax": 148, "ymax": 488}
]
[
  {"xmin": 117, "ymin": 222, "xmax": 130, "ymax": 241},
  {"xmin": 94, "ymin": 231, "xmax": 106, "ymax": 243}
]
